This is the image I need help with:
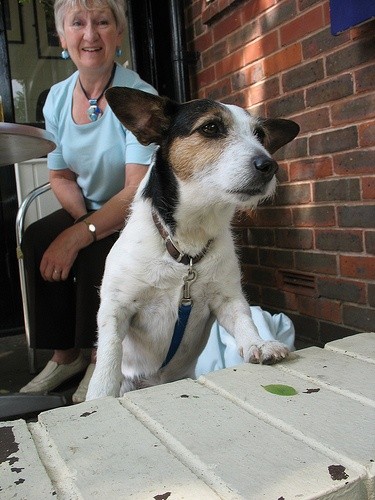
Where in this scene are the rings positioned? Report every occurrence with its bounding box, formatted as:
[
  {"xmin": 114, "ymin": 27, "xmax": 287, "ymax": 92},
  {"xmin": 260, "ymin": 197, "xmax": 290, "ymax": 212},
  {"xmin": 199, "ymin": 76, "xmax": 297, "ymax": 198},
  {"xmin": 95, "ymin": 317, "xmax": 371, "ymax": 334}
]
[{"xmin": 53, "ymin": 270, "xmax": 62, "ymax": 274}]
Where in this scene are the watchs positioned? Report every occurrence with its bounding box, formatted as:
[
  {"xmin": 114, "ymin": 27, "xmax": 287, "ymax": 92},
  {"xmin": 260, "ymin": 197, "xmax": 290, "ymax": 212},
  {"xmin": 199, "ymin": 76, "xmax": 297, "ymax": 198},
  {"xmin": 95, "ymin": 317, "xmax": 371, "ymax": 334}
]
[{"xmin": 84, "ymin": 220, "xmax": 96, "ymax": 242}]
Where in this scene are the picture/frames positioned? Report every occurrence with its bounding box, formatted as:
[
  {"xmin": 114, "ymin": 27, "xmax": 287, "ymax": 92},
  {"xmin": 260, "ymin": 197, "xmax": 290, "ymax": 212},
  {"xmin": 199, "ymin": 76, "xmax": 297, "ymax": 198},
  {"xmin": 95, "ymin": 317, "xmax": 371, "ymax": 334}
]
[{"xmin": 33, "ymin": 0, "xmax": 72, "ymax": 59}]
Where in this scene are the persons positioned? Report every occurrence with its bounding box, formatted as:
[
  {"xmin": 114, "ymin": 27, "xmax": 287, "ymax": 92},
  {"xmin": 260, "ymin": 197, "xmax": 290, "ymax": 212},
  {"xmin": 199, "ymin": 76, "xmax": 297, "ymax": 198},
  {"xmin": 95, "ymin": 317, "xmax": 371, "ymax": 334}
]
[{"xmin": 19, "ymin": 0, "xmax": 160, "ymax": 404}]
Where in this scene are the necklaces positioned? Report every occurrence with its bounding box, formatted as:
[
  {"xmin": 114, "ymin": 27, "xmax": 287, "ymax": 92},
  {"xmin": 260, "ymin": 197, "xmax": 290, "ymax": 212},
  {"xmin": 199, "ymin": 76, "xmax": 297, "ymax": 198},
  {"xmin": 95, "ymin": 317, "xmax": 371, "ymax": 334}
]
[{"xmin": 78, "ymin": 62, "xmax": 116, "ymax": 122}]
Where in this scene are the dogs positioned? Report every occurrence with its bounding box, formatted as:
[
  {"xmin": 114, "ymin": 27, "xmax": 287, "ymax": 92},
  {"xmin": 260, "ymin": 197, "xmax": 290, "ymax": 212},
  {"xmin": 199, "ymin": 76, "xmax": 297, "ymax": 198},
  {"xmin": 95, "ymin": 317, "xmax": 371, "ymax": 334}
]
[{"xmin": 84, "ymin": 85, "xmax": 301, "ymax": 402}]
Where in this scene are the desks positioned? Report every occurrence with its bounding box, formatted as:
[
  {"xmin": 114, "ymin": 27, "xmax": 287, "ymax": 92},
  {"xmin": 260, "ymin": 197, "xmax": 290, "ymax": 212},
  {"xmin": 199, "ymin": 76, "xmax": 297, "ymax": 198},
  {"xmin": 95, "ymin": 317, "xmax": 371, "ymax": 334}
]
[{"xmin": 0, "ymin": 122, "xmax": 57, "ymax": 167}]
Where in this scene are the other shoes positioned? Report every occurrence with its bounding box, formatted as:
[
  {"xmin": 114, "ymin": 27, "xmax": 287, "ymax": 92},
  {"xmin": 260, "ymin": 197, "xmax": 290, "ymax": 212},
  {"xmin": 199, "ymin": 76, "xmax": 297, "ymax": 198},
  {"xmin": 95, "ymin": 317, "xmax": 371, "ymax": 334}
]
[
  {"xmin": 20, "ymin": 351, "xmax": 86, "ymax": 394},
  {"xmin": 72, "ymin": 363, "xmax": 95, "ymax": 403}
]
